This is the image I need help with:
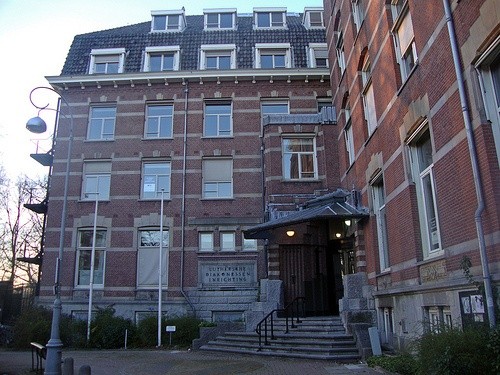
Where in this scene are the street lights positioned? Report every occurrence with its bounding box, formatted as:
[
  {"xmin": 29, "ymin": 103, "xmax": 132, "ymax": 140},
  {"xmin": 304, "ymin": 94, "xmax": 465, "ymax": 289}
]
[{"xmin": 26, "ymin": 86, "xmax": 75, "ymax": 375}]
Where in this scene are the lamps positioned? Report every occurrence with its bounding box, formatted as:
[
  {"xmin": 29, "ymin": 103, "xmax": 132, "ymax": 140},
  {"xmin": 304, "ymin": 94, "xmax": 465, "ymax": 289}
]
[
  {"xmin": 345, "ymin": 219, "xmax": 352, "ymax": 226},
  {"xmin": 336, "ymin": 233, "xmax": 342, "ymax": 238},
  {"xmin": 286, "ymin": 230, "xmax": 296, "ymax": 238}
]
